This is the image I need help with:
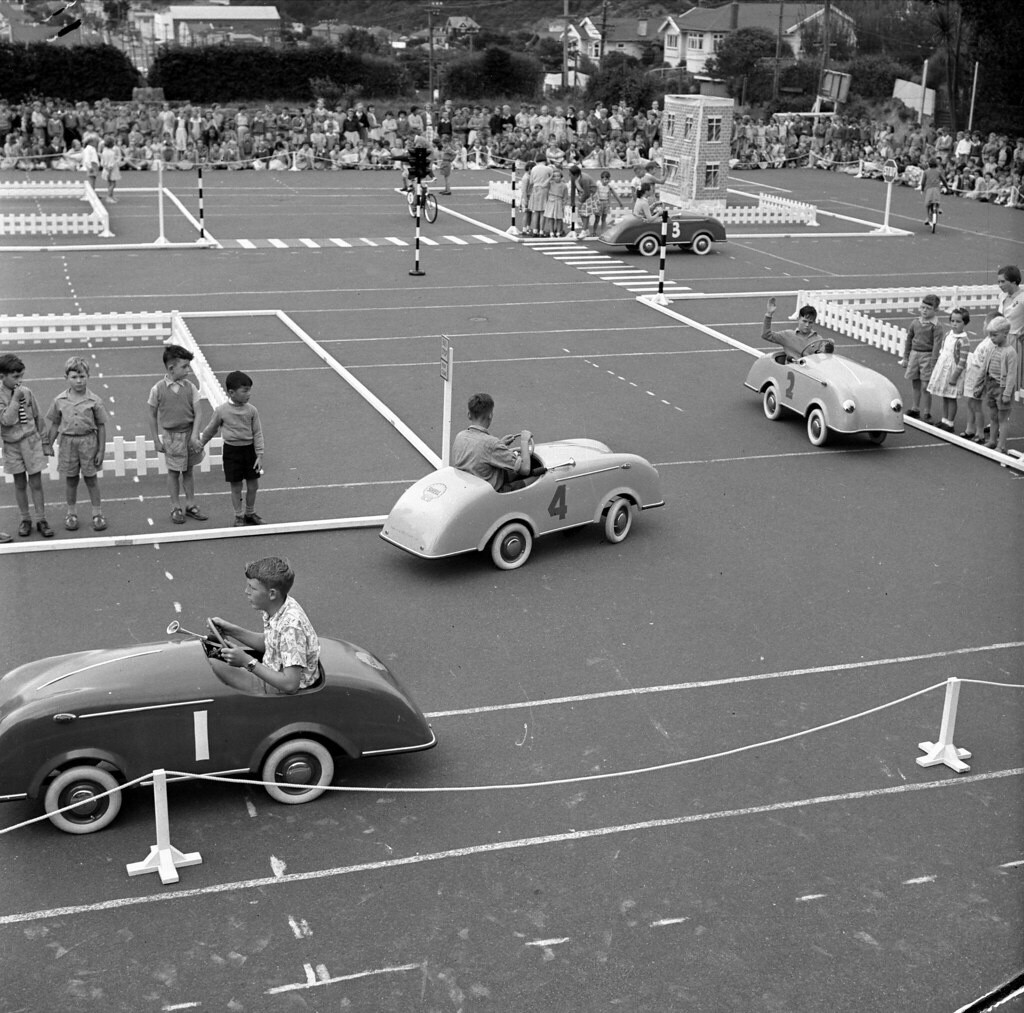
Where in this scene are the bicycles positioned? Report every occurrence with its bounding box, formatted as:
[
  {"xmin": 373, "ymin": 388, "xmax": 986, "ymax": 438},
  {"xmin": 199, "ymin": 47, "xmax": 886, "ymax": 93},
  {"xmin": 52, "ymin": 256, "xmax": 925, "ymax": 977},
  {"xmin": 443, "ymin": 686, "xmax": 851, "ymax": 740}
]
[
  {"xmin": 406, "ymin": 176, "xmax": 439, "ymax": 224},
  {"xmin": 927, "ymin": 203, "xmax": 939, "ymax": 232}
]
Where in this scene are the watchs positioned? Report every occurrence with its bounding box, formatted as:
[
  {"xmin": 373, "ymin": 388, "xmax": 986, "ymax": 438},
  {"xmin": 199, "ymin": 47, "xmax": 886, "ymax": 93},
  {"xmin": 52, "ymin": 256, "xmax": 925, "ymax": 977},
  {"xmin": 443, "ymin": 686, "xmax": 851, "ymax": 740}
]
[{"xmin": 247, "ymin": 658, "xmax": 258, "ymax": 672}]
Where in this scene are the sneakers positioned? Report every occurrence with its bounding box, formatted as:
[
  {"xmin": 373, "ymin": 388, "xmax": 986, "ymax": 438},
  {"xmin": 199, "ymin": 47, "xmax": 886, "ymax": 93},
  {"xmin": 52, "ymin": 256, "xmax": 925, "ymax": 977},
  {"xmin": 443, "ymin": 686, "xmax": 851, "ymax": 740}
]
[
  {"xmin": 92, "ymin": 514, "xmax": 107, "ymax": 530},
  {"xmin": 170, "ymin": 506, "xmax": 185, "ymax": 524},
  {"xmin": 578, "ymin": 228, "xmax": 596, "ymax": 238},
  {"xmin": 959, "ymin": 431, "xmax": 974, "ymax": 438},
  {"xmin": 234, "ymin": 515, "xmax": 244, "ymax": 525},
  {"xmin": 244, "ymin": 513, "xmax": 266, "ymax": 525},
  {"xmin": 185, "ymin": 503, "xmax": 210, "ymax": 521},
  {"xmin": 972, "ymin": 434, "xmax": 986, "ymax": 444},
  {"xmin": 38, "ymin": 521, "xmax": 54, "ymax": 536},
  {"xmin": 18, "ymin": 520, "xmax": 32, "ymax": 537},
  {"xmin": 64, "ymin": 513, "xmax": 79, "ymax": 530}
]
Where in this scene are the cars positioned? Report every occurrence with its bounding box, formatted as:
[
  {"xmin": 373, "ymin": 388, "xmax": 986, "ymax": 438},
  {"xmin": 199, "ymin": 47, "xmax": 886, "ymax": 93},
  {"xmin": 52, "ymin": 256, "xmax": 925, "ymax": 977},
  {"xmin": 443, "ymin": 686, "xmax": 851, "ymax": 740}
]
[
  {"xmin": 377, "ymin": 437, "xmax": 664, "ymax": 569},
  {"xmin": 0, "ymin": 616, "xmax": 436, "ymax": 834},
  {"xmin": 742, "ymin": 339, "xmax": 907, "ymax": 448},
  {"xmin": 598, "ymin": 203, "xmax": 727, "ymax": 256}
]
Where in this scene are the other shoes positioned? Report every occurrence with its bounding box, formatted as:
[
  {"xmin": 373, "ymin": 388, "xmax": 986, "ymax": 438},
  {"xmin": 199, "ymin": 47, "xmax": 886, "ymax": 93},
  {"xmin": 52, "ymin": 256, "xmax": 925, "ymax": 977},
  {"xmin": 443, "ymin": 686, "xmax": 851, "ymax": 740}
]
[
  {"xmin": 438, "ymin": 191, "xmax": 452, "ymax": 196},
  {"xmin": 935, "ymin": 418, "xmax": 954, "ymax": 433},
  {"xmin": 0, "ymin": 532, "xmax": 13, "ymax": 543},
  {"xmin": 923, "ymin": 412, "xmax": 935, "ymax": 425},
  {"xmin": 522, "ymin": 228, "xmax": 564, "ymax": 237},
  {"xmin": 904, "ymin": 409, "xmax": 920, "ymax": 419},
  {"xmin": 400, "ymin": 187, "xmax": 408, "ymax": 192},
  {"xmin": 995, "ymin": 446, "xmax": 1006, "ymax": 453},
  {"xmin": 985, "ymin": 441, "xmax": 995, "ymax": 448}
]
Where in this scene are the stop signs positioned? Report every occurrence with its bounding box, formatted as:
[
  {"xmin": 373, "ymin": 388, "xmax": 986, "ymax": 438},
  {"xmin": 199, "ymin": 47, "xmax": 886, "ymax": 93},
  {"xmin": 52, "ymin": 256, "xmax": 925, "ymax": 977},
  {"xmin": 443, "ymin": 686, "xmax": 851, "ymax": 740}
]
[{"xmin": 881, "ymin": 159, "xmax": 897, "ymax": 183}]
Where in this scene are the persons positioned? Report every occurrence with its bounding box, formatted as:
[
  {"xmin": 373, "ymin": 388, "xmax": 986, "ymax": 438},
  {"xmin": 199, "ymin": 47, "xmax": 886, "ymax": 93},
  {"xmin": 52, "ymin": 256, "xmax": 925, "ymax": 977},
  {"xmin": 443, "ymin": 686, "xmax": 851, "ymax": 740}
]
[
  {"xmin": 519, "ymin": 155, "xmax": 624, "ymax": 240},
  {"xmin": 206, "ymin": 555, "xmax": 321, "ymax": 694},
  {"xmin": 730, "ymin": 111, "xmax": 930, "ymax": 188},
  {"xmin": 199, "ymin": 370, "xmax": 267, "ymax": 526},
  {"xmin": 439, "ymin": 133, "xmax": 457, "ymax": 195},
  {"xmin": 958, "ymin": 311, "xmax": 1005, "ymax": 444},
  {"xmin": 81, "ymin": 134, "xmax": 122, "ymax": 202},
  {"xmin": 900, "ymin": 293, "xmax": 944, "ymax": 422},
  {"xmin": 630, "ymin": 160, "xmax": 669, "ymax": 222},
  {"xmin": 249, "ymin": 97, "xmax": 610, "ymax": 169},
  {"xmin": 921, "ymin": 158, "xmax": 950, "ymax": 225},
  {"xmin": 973, "ymin": 316, "xmax": 1019, "ymax": 453},
  {"xmin": 924, "ymin": 123, "xmax": 1024, "ymax": 209},
  {"xmin": 761, "ymin": 297, "xmax": 825, "ymax": 365},
  {"xmin": 0, "ymin": 354, "xmax": 55, "ymax": 537},
  {"xmin": 45, "ymin": 355, "xmax": 108, "ymax": 531},
  {"xmin": 0, "ymin": 97, "xmax": 246, "ymax": 172},
  {"xmin": 983, "ymin": 264, "xmax": 1024, "ymax": 435},
  {"xmin": 386, "ymin": 135, "xmax": 437, "ymax": 191},
  {"xmin": 611, "ymin": 99, "xmax": 666, "ymax": 167},
  {"xmin": 452, "ymin": 393, "xmax": 539, "ymax": 492},
  {"xmin": 926, "ymin": 308, "xmax": 970, "ymax": 432},
  {"xmin": 147, "ymin": 343, "xmax": 209, "ymax": 523}
]
